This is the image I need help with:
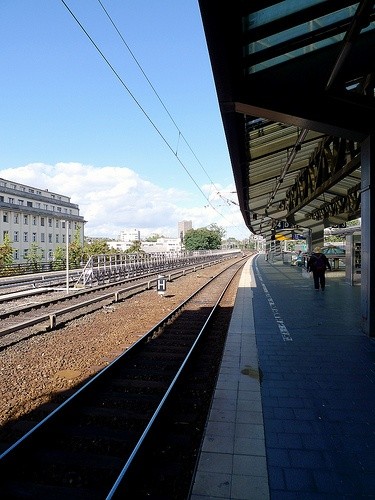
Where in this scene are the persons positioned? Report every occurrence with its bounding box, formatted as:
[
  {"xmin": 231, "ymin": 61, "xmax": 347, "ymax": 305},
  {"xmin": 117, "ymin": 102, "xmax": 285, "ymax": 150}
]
[{"xmin": 306, "ymin": 247, "xmax": 331, "ymax": 292}]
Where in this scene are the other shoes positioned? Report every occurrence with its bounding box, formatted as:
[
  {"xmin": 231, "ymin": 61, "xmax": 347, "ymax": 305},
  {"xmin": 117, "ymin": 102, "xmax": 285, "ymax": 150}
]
[
  {"xmin": 323, "ymin": 289, "xmax": 325, "ymax": 291},
  {"xmin": 315, "ymin": 289, "xmax": 319, "ymax": 291}
]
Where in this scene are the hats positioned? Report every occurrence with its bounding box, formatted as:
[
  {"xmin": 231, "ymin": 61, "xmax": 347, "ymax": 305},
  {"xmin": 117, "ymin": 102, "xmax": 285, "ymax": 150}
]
[{"xmin": 313, "ymin": 247, "xmax": 321, "ymax": 253}]
